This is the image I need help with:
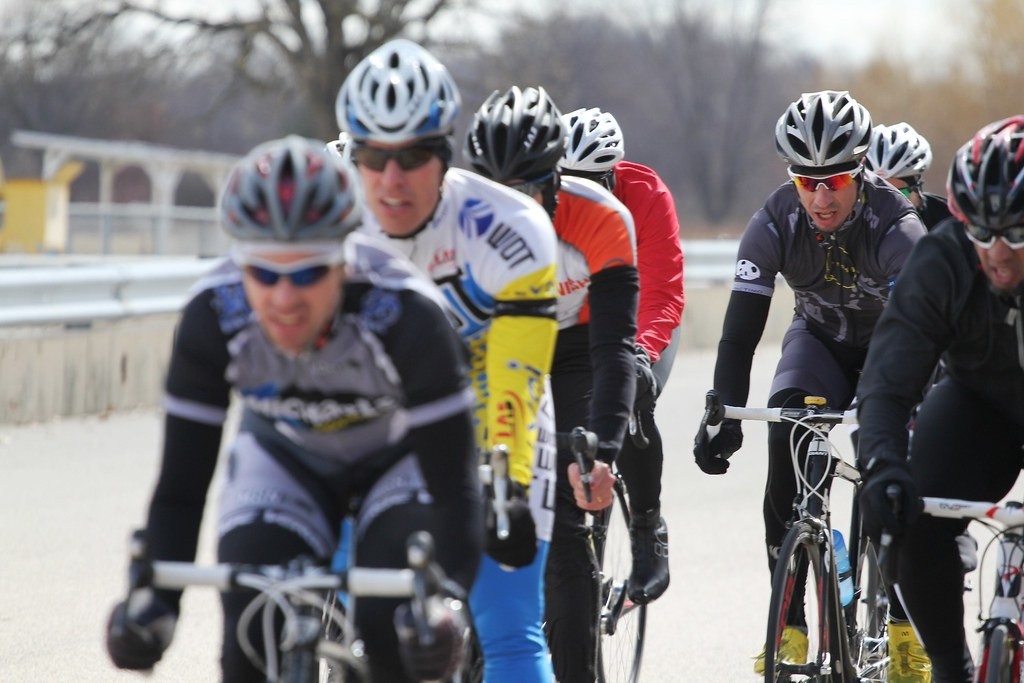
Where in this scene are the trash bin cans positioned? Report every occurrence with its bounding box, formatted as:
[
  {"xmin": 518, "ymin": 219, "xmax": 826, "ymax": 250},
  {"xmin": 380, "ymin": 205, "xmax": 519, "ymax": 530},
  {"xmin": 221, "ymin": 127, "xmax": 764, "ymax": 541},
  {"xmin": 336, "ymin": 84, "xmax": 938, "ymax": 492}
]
[{"xmin": 0, "ymin": 156, "xmax": 83, "ymax": 255}]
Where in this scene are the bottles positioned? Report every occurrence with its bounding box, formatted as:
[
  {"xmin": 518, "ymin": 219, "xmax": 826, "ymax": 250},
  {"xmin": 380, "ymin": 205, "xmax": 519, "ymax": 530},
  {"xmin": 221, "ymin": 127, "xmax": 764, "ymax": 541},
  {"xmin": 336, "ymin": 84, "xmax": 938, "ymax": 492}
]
[{"xmin": 833, "ymin": 530, "xmax": 854, "ymax": 606}]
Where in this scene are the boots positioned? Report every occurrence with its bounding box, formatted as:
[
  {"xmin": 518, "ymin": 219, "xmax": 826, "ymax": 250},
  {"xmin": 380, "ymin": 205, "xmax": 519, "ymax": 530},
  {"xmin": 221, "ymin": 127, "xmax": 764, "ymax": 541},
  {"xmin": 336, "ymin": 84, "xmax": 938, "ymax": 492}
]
[{"xmin": 627, "ymin": 500, "xmax": 670, "ymax": 604}]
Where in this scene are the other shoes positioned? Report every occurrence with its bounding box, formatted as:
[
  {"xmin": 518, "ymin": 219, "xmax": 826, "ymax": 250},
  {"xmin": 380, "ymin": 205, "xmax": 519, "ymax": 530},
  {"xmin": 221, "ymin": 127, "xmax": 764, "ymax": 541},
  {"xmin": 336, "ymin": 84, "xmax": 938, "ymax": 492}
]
[{"xmin": 956, "ymin": 536, "xmax": 978, "ymax": 570}]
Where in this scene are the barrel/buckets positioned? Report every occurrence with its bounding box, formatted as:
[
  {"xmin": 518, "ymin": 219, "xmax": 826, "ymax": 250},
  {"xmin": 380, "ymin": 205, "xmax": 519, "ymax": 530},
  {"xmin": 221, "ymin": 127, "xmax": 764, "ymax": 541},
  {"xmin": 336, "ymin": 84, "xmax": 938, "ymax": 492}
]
[{"xmin": 1, "ymin": 179, "xmax": 69, "ymax": 254}]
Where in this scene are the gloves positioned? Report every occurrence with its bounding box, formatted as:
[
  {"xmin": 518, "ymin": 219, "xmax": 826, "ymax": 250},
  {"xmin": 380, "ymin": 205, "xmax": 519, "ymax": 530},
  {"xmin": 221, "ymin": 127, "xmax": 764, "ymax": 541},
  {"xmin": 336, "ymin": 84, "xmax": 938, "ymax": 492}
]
[
  {"xmin": 693, "ymin": 391, "xmax": 743, "ymax": 475},
  {"xmin": 107, "ymin": 587, "xmax": 179, "ymax": 669},
  {"xmin": 395, "ymin": 599, "xmax": 458, "ymax": 678},
  {"xmin": 635, "ymin": 344, "xmax": 658, "ymax": 404},
  {"xmin": 864, "ymin": 461, "xmax": 924, "ymax": 520}
]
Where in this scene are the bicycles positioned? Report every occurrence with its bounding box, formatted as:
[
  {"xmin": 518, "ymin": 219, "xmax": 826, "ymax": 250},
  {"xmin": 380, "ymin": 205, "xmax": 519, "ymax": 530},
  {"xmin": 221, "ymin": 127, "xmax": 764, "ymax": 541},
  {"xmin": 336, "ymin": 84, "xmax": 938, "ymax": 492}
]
[
  {"xmin": 693, "ymin": 388, "xmax": 922, "ymax": 683},
  {"xmin": 872, "ymin": 481, "xmax": 1023, "ymax": 683},
  {"xmin": 125, "ymin": 370, "xmax": 650, "ymax": 683}
]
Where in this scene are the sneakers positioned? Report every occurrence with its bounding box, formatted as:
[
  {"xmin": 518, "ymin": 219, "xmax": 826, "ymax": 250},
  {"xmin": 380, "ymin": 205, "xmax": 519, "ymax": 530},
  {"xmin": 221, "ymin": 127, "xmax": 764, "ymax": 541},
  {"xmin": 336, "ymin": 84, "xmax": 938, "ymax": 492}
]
[
  {"xmin": 886, "ymin": 622, "xmax": 933, "ymax": 683},
  {"xmin": 749, "ymin": 628, "xmax": 809, "ymax": 673}
]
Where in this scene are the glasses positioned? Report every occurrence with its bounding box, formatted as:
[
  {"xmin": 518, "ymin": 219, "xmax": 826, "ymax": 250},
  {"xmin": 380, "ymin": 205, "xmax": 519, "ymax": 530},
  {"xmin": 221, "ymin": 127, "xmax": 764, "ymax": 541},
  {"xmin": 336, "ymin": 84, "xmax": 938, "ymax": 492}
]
[
  {"xmin": 787, "ymin": 164, "xmax": 864, "ymax": 191},
  {"xmin": 964, "ymin": 222, "xmax": 1024, "ymax": 249},
  {"xmin": 350, "ymin": 146, "xmax": 444, "ymax": 172},
  {"xmin": 508, "ymin": 172, "xmax": 553, "ymax": 196},
  {"xmin": 236, "ymin": 256, "xmax": 333, "ymax": 286},
  {"xmin": 896, "ymin": 184, "xmax": 920, "ymax": 198}
]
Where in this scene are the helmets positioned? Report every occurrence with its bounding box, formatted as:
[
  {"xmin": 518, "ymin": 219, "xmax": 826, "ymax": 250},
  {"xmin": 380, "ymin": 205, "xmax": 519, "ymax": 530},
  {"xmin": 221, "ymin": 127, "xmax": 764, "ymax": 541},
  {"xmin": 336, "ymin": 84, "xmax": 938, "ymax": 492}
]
[
  {"xmin": 775, "ymin": 89, "xmax": 872, "ymax": 174},
  {"xmin": 219, "ymin": 135, "xmax": 363, "ymax": 242},
  {"xmin": 462, "ymin": 86, "xmax": 562, "ymax": 182},
  {"xmin": 335, "ymin": 39, "xmax": 461, "ymax": 142},
  {"xmin": 948, "ymin": 115, "xmax": 1024, "ymax": 228},
  {"xmin": 865, "ymin": 122, "xmax": 932, "ymax": 179},
  {"xmin": 555, "ymin": 107, "xmax": 625, "ymax": 172}
]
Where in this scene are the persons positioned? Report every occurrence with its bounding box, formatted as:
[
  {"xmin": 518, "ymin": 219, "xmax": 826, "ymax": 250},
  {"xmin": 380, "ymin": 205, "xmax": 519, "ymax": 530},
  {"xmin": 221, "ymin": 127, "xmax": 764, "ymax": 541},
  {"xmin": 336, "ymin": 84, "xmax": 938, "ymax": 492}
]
[
  {"xmin": 465, "ymin": 86, "xmax": 640, "ymax": 683},
  {"xmin": 556, "ymin": 108, "xmax": 684, "ymax": 604},
  {"xmin": 335, "ymin": 41, "xmax": 556, "ymax": 683},
  {"xmin": 108, "ymin": 137, "xmax": 485, "ymax": 683},
  {"xmin": 693, "ymin": 91, "xmax": 1024, "ymax": 683}
]
